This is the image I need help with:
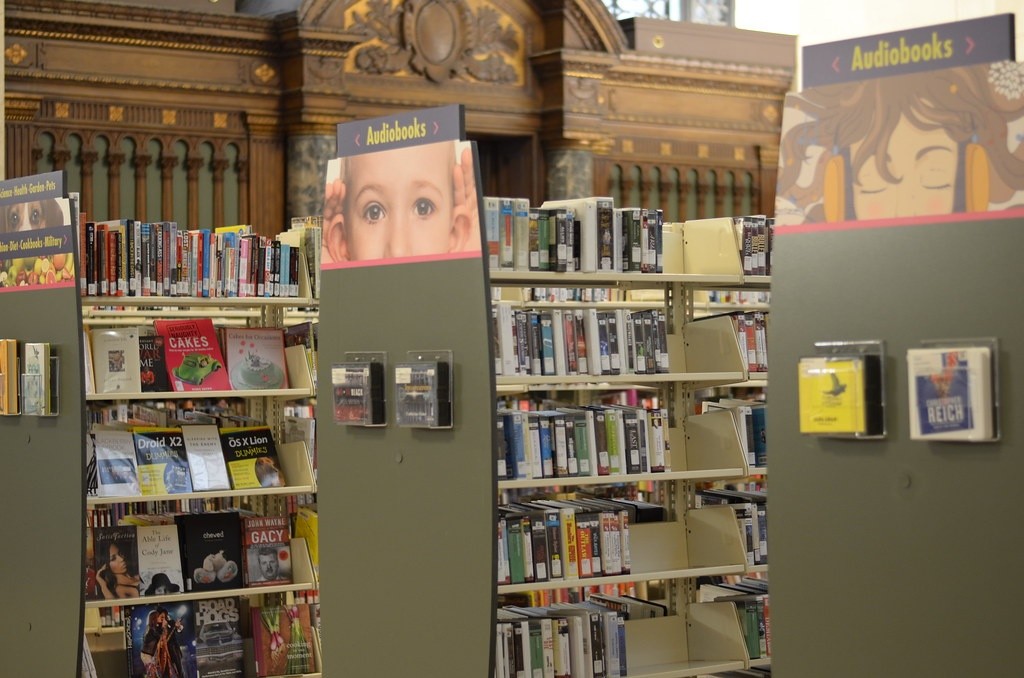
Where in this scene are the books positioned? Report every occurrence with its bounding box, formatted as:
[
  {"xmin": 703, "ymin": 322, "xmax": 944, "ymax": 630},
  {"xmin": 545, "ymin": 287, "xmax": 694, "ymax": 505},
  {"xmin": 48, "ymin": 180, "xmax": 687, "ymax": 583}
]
[
  {"xmin": 24, "ymin": 342, "xmax": 52, "ymax": 417},
  {"xmin": 1, "ymin": 338, "xmax": 20, "ymax": 415},
  {"xmin": 62, "ymin": 188, "xmax": 772, "ymax": 677}
]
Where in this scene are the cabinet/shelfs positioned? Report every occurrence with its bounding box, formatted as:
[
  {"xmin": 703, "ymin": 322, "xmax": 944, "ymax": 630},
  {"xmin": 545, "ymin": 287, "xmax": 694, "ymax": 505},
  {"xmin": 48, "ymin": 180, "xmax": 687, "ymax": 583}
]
[
  {"xmin": 765, "ymin": 13, "xmax": 1023, "ymax": 678},
  {"xmin": 0, "ymin": 169, "xmax": 321, "ymax": 678},
  {"xmin": 316, "ymin": 102, "xmax": 749, "ymax": 678},
  {"xmin": 683, "ymin": 217, "xmax": 771, "ymax": 678}
]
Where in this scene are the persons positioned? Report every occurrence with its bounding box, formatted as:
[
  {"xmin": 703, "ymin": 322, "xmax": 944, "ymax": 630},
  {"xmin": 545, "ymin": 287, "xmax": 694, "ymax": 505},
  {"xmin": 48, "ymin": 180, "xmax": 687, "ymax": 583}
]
[
  {"xmin": 95, "ymin": 542, "xmax": 141, "ymax": 599},
  {"xmin": 322, "ymin": 141, "xmax": 480, "ymax": 267},
  {"xmin": 145, "ymin": 572, "xmax": 179, "ymax": 597},
  {"xmin": 255, "ymin": 545, "xmax": 286, "ymax": 581},
  {"xmin": 141, "ymin": 607, "xmax": 184, "ymax": 678}
]
